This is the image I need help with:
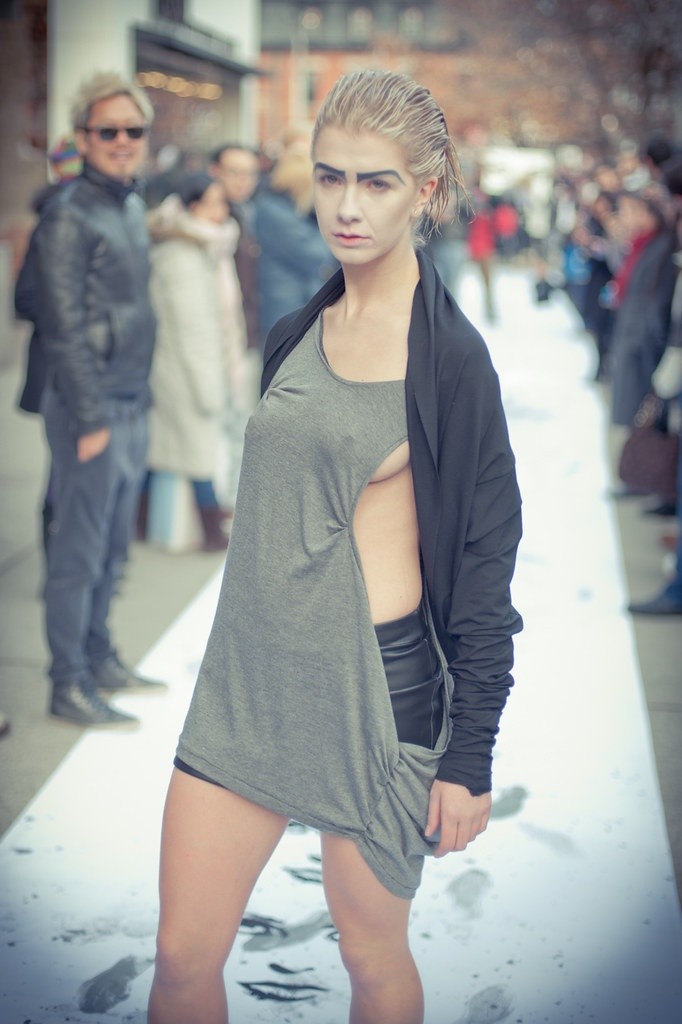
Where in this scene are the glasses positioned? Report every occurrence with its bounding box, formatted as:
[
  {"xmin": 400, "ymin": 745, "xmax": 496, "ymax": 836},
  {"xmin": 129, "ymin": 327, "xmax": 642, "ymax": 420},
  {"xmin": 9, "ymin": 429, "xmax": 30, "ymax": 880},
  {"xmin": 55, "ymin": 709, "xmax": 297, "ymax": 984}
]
[{"xmin": 80, "ymin": 127, "xmax": 149, "ymax": 139}]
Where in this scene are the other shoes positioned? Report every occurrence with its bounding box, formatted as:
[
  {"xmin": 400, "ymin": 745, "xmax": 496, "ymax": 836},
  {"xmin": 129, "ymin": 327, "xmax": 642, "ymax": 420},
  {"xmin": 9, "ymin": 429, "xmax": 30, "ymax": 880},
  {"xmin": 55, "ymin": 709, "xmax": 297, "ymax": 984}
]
[{"xmin": 629, "ymin": 592, "xmax": 681, "ymax": 616}]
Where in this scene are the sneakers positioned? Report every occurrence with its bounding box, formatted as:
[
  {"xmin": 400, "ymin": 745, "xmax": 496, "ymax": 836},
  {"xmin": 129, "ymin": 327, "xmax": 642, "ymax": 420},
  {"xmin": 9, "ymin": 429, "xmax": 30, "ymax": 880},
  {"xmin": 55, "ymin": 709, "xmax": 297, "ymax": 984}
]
[
  {"xmin": 48, "ymin": 679, "xmax": 140, "ymax": 731},
  {"xmin": 87, "ymin": 654, "xmax": 166, "ymax": 695}
]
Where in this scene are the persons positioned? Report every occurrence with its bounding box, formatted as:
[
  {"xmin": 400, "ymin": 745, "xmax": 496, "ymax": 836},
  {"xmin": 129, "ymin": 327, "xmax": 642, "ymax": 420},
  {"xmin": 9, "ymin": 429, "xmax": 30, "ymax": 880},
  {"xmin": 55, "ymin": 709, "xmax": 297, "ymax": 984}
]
[
  {"xmin": 147, "ymin": 70, "xmax": 525, "ymax": 1024},
  {"xmin": 198, "ymin": 144, "xmax": 527, "ymax": 362},
  {"xmin": 547, "ymin": 138, "xmax": 682, "ymax": 615},
  {"xmin": 15, "ymin": 74, "xmax": 166, "ymax": 732},
  {"xmin": 130, "ymin": 169, "xmax": 253, "ymax": 558}
]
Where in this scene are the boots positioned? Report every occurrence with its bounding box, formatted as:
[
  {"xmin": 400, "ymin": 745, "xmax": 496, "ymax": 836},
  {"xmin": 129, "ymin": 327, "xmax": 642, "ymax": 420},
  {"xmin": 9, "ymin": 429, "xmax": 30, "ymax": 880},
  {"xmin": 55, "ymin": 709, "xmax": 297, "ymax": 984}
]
[
  {"xmin": 137, "ymin": 495, "xmax": 147, "ymax": 541},
  {"xmin": 202, "ymin": 505, "xmax": 229, "ymax": 553}
]
